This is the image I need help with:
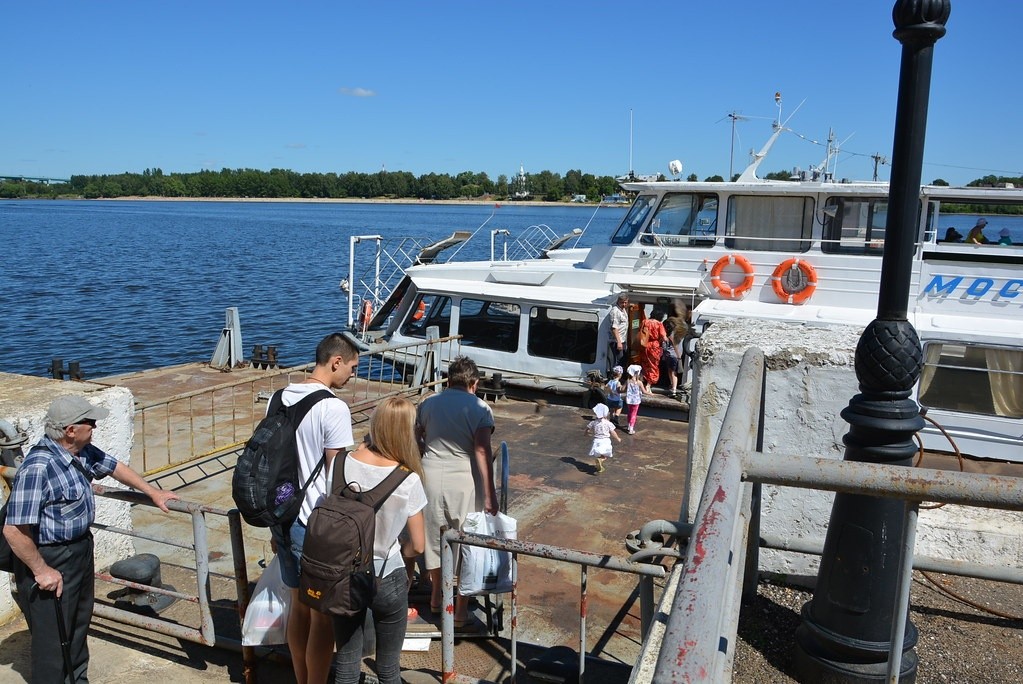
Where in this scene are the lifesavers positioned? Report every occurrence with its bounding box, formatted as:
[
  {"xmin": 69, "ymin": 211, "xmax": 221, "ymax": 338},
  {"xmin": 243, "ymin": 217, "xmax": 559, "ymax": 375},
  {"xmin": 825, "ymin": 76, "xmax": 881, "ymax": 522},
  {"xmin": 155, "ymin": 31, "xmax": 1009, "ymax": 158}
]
[
  {"xmin": 709, "ymin": 253, "xmax": 755, "ymax": 299},
  {"xmin": 358, "ymin": 300, "xmax": 373, "ymax": 331},
  {"xmin": 770, "ymin": 258, "xmax": 817, "ymax": 305},
  {"xmin": 396, "ymin": 296, "xmax": 425, "ymax": 322}
]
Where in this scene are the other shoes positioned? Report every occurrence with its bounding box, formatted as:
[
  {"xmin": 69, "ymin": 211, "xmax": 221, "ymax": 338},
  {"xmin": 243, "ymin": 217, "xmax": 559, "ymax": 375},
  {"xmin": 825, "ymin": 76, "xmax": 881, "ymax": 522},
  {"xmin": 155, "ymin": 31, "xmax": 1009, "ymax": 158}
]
[
  {"xmin": 595, "ymin": 458, "xmax": 605, "ymax": 472},
  {"xmin": 627, "ymin": 427, "xmax": 636, "ymax": 435},
  {"xmin": 645, "ymin": 386, "xmax": 652, "ymax": 393},
  {"xmin": 668, "ymin": 390, "xmax": 676, "ymax": 397},
  {"xmin": 408, "ymin": 607, "xmax": 417, "ymax": 619}
]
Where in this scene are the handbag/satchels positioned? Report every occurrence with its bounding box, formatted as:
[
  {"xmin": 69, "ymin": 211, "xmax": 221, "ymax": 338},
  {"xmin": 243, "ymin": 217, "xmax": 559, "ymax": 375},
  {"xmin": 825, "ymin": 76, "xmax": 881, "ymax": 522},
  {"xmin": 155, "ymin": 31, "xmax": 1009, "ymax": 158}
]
[
  {"xmin": 241, "ymin": 554, "xmax": 295, "ymax": 647},
  {"xmin": 0, "ymin": 499, "xmax": 18, "ymax": 572},
  {"xmin": 458, "ymin": 507, "xmax": 517, "ymax": 596},
  {"xmin": 662, "ymin": 339, "xmax": 677, "ymax": 364}
]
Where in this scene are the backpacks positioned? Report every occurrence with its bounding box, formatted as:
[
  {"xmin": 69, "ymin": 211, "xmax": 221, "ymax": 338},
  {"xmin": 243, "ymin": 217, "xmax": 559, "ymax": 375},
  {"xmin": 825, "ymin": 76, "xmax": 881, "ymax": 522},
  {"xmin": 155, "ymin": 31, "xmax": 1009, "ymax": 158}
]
[
  {"xmin": 297, "ymin": 453, "xmax": 415, "ymax": 617},
  {"xmin": 232, "ymin": 388, "xmax": 341, "ymax": 528}
]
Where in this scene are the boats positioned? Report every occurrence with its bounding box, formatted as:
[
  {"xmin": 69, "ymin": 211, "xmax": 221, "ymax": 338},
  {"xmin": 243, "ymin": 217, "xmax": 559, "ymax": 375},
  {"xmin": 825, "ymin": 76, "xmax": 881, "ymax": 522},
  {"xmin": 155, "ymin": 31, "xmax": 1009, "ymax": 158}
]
[{"xmin": 340, "ymin": 94, "xmax": 1023, "ymax": 463}]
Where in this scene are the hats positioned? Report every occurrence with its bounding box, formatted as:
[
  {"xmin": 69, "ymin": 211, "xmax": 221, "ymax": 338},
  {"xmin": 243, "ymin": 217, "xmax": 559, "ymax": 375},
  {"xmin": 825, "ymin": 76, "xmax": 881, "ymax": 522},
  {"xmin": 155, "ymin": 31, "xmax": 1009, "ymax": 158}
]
[{"xmin": 47, "ymin": 396, "xmax": 110, "ymax": 427}]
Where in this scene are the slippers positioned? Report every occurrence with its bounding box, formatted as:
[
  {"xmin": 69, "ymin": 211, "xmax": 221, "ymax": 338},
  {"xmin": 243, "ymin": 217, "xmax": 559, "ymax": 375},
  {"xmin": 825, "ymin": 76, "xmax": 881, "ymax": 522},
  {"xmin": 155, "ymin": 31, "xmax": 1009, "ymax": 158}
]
[
  {"xmin": 454, "ymin": 610, "xmax": 478, "ymax": 627},
  {"xmin": 431, "ymin": 604, "xmax": 442, "ymax": 612}
]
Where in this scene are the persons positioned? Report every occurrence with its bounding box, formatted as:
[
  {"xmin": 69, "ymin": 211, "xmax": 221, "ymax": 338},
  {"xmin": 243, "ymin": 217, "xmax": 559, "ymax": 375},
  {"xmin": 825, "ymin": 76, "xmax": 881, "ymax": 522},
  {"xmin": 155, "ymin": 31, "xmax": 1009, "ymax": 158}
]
[
  {"xmin": 266, "ymin": 333, "xmax": 360, "ymax": 684},
  {"xmin": 965, "ymin": 218, "xmax": 987, "ymax": 245},
  {"xmin": 584, "ymin": 403, "xmax": 621, "ymax": 473},
  {"xmin": 608, "ymin": 293, "xmax": 630, "ymax": 385},
  {"xmin": 998, "ymin": 228, "xmax": 1012, "ymax": 246},
  {"xmin": 326, "ymin": 397, "xmax": 428, "ymax": 684},
  {"xmin": 2, "ymin": 395, "xmax": 181, "ymax": 684},
  {"xmin": 604, "ymin": 365, "xmax": 654, "ymax": 435},
  {"xmin": 944, "ymin": 228, "xmax": 962, "ymax": 243},
  {"xmin": 418, "ymin": 357, "xmax": 499, "ymax": 628},
  {"xmin": 638, "ymin": 308, "xmax": 684, "ymax": 397}
]
